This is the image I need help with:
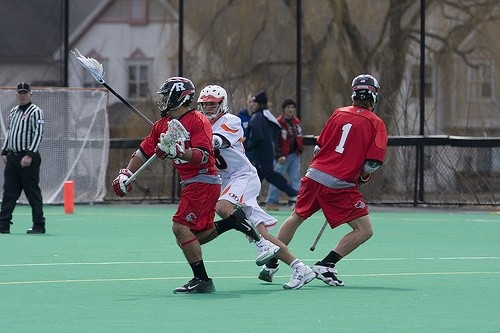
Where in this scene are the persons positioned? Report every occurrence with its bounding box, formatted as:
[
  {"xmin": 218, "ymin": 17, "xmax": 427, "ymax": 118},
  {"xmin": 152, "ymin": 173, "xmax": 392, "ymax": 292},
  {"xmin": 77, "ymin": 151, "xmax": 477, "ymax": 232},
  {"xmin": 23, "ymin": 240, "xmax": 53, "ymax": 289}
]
[
  {"xmin": 246, "ymin": 91, "xmax": 300, "ymax": 208},
  {"xmin": 235, "ymin": 92, "xmax": 257, "ymax": 159},
  {"xmin": 195, "ymin": 84, "xmax": 316, "ymax": 290},
  {"xmin": 266, "ymin": 98, "xmax": 303, "ymax": 211},
  {"xmin": 112, "ymin": 76, "xmax": 261, "ymax": 294},
  {"xmin": 258, "ymin": 74, "xmax": 388, "ymax": 287},
  {"xmin": 1, "ymin": 81, "xmax": 47, "ymax": 234}
]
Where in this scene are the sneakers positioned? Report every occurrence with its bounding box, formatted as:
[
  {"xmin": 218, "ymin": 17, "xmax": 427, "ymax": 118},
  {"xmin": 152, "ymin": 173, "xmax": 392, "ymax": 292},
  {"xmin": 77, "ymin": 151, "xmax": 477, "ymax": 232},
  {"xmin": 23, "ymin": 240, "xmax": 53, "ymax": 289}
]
[
  {"xmin": 311, "ymin": 261, "xmax": 344, "ymax": 287},
  {"xmin": 255, "ymin": 239, "xmax": 280, "ymax": 266},
  {"xmin": 173, "ymin": 277, "xmax": 216, "ymax": 294},
  {"xmin": 283, "ymin": 264, "xmax": 316, "ymax": 290},
  {"xmin": 230, "ymin": 205, "xmax": 260, "ymax": 242},
  {"xmin": 258, "ymin": 264, "xmax": 280, "ymax": 283}
]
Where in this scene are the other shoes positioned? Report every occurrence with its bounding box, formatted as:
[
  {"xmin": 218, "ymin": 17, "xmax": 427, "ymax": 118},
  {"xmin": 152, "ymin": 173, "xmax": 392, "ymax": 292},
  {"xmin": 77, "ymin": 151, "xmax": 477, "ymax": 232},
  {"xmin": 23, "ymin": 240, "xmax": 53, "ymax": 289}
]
[
  {"xmin": 27, "ymin": 226, "xmax": 45, "ymax": 234},
  {"xmin": 286, "ymin": 200, "xmax": 296, "ymax": 207},
  {"xmin": 0, "ymin": 229, "xmax": 10, "ymax": 233},
  {"xmin": 266, "ymin": 204, "xmax": 279, "ymax": 211}
]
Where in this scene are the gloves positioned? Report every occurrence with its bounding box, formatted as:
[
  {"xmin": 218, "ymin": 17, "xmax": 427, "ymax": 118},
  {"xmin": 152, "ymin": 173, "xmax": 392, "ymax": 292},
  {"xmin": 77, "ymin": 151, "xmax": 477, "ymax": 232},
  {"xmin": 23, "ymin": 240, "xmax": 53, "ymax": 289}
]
[
  {"xmin": 112, "ymin": 169, "xmax": 136, "ymax": 197},
  {"xmin": 154, "ymin": 133, "xmax": 185, "ymax": 159}
]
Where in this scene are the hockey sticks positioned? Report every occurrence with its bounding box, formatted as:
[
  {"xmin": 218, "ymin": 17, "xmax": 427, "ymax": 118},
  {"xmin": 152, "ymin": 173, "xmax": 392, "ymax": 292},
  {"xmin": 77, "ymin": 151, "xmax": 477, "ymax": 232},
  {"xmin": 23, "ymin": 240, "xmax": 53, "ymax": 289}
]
[
  {"xmin": 76, "ymin": 54, "xmax": 155, "ymax": 127},
  {"xmin": 310, "ymin": 220, "xmax": 328, "ymax": 251},
  {"xmin": 123, "ymin": 119, "xmax": 189, "ymax": 187}
]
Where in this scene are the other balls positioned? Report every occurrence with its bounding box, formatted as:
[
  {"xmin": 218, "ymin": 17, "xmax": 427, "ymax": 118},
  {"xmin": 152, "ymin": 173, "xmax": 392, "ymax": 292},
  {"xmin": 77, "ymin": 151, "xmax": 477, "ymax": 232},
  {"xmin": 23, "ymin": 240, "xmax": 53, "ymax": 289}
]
[{"xmin": 163, "ymin": 134, "xmax": 171, "ymax": 143}]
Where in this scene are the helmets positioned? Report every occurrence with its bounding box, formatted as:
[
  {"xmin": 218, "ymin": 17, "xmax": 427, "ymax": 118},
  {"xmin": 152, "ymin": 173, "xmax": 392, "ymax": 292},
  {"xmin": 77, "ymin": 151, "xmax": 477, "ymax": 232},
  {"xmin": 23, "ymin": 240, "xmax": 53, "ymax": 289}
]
[
  {"xmin": 158, "ymin": 77, "xmax": 195, "ymax": 118},
  {"xmin": 351, "ymin": 74, "xmax": 380, "ymax": 103},
  {"xmin": 196, "ymin": 85, "xmax": 228, "ymax": 123}
]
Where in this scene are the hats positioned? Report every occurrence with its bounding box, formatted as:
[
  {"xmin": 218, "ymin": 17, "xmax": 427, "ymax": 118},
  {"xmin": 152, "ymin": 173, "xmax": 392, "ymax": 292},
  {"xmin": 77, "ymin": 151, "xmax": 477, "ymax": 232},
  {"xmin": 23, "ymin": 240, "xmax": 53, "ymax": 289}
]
[
  {"xmin": 253, "ymin": 91, "xmax": 268, "ymax": 105},
  {"xmin": 16, "ymin": 81, "xmax": 31, "ymax": 93}
]
